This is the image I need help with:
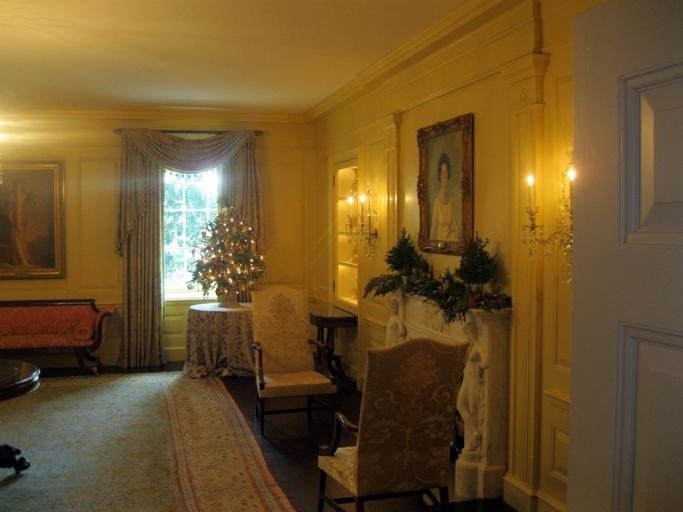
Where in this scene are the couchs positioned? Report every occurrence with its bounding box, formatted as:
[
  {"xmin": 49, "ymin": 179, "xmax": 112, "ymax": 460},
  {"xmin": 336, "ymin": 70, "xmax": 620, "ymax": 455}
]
[{"xmin": 0, "ymin": 298, "xmax": 114, "ymax": 377}]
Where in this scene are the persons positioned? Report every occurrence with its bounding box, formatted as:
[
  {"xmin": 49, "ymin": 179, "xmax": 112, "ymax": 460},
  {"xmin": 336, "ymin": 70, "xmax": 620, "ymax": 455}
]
[
  {"xmin": 456, "ymin": 322, "xmax": 489, "ymax": 451},
  {"xmin": 385, "ymin": 299, "xmax": 406, "ymax": 348},
  {"xmin": 428, "ymin": 153, "xmax": 461, "ymax": 241}
]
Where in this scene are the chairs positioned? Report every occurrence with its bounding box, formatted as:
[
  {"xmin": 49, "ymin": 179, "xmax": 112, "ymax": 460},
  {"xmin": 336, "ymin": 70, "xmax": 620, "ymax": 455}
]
[{"xmin": 315, "ymin": 337, "xmax": 472, "ymax": 511}]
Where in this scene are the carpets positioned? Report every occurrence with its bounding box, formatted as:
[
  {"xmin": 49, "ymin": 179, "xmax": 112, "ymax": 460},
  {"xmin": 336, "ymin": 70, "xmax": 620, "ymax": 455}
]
[{"xmin": 0, "ymin": 370, "xmax": 298, "ymax": 512}]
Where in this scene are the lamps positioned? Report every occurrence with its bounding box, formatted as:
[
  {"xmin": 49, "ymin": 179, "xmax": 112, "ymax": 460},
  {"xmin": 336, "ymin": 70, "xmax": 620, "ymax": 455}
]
[
  {"xmin": 340, "ymin": 183, "xmax": 378, "ymax": 266},
  {"xmin": 522, "ymin": 162, "xmax": 577, "ymax": 280}
]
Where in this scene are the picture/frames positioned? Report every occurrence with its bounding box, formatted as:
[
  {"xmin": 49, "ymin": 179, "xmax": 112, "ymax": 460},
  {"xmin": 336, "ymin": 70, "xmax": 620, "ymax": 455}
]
[
  {"xmin": 415, "ymin": 110, "xmax": 476, "ymax": 256},
  {"xmin": 249, "ymin": 285, "xmax": 339, "ymax": 442},
  {"xmin": 0, "ymin": 159, "xmax": 68, "ymax": 281}
]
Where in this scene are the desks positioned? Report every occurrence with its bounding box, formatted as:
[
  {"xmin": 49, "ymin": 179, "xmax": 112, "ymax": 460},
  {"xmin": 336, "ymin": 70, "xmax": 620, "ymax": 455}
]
[
  {"xmin": 182, "ymin": 299, "xmax": 253, "ymax": 378},
  {"xmin": 0, "ymin": 359, "xmax": 42, "ymax": 474}
]
